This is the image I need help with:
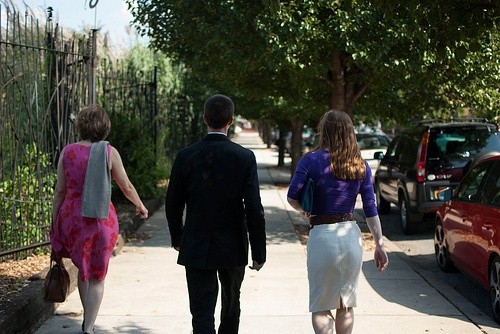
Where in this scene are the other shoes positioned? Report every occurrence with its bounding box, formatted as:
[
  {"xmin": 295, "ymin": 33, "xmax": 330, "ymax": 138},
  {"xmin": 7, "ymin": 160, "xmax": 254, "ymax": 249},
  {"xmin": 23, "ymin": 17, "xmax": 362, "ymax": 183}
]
[{"xmin": 82, "ymin": 319, "xmax": 94, "ymax": 332}]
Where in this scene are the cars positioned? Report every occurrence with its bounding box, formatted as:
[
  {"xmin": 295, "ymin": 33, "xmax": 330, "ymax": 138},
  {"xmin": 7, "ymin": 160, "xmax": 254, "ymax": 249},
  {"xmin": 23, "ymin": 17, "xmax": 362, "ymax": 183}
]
[
  {"xmin": 434, "ymin": 152, "xmax": 500, "ymax": 325},
  {"xmin": 354, "ymin": 133, "xmax": 392, "ymax": 184}
]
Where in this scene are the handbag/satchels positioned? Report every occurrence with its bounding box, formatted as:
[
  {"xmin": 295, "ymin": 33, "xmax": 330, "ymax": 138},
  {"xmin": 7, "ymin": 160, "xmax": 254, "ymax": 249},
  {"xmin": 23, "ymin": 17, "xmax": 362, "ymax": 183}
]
[{"xmin": 43, "ymin": 251, "xmax": 70, "ymax": 304}]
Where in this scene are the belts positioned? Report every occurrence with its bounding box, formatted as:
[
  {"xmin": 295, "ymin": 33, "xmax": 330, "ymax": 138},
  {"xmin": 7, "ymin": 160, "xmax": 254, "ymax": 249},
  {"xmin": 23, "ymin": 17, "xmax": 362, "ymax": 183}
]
[{"xmin": 309, "ymin": 213, "xmax": 354, "ymax": 225}]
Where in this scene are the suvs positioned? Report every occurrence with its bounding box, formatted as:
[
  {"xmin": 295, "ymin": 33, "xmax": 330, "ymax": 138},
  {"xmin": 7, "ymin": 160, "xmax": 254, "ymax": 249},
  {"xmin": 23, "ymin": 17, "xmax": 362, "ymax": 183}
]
[{"xmin": 373, "ymin": 118, "xmax": 499, "ymax": 234}]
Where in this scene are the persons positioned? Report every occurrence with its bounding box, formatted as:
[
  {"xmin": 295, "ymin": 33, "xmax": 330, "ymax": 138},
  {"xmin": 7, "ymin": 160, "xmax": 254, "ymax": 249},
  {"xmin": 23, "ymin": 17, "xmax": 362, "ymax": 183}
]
[
  {"xmin": 287, "ymin": 109, "xmax": 389, "ymax": 334},
  {"xmin": 165, "ymin": 94, "xmax": 266, "ymax": 334},
  {"xmin": 49, "ymin": 105, "xmax": 149, "ymax": 334}
]
